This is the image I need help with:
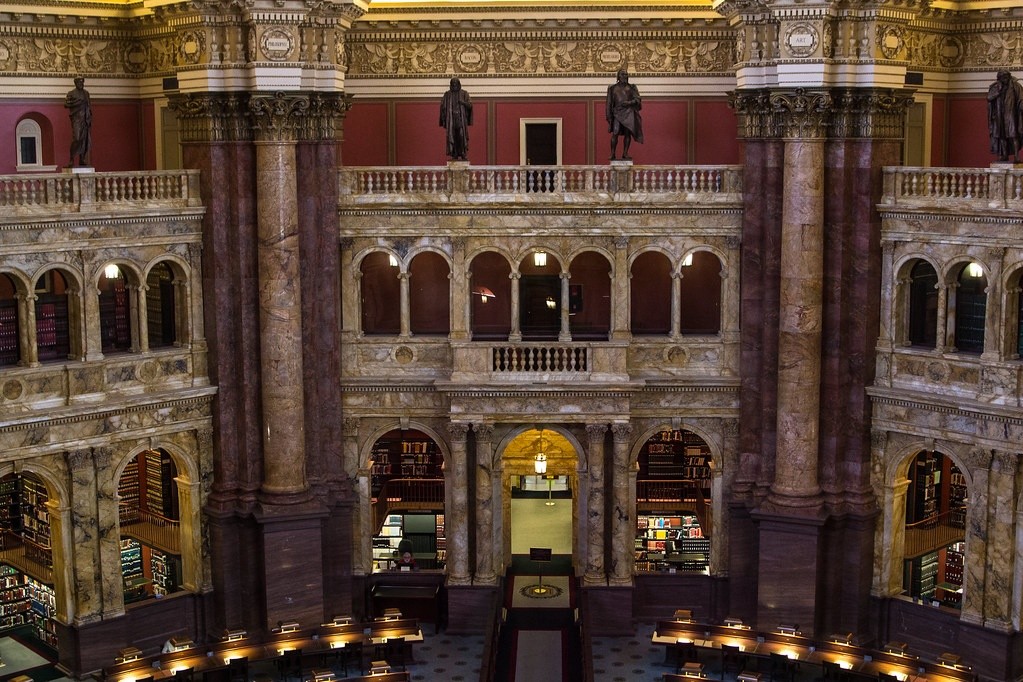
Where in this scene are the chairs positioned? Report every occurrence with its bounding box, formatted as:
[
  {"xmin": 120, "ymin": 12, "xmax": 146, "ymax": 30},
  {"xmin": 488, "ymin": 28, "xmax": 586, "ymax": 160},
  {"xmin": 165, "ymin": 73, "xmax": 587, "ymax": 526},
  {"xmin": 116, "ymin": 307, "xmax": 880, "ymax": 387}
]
[
  {"xmin": 399, "ymin": 540, "xmax": 413, "ymax": 552},
  {"xmin": 136, "ymin": 675, "xmax": 154, "ymax": 682},
  {"xmin": 676, "ymin": 641, "xmax": 697, "ymax": 673},
  {"xmin": 823, "ymin": 660, "xmax": 841, "ymax": 682},
  {"xmin": 769, "ymin": 651, "xmax": 796, "ymax": 682},
  {"xmin": 378, "ymin": 637, "xmax": 406, "ymax": 672},
  {"xmin": 340, "ymin": 642, "xmax": 364, "ymax": 677},
  {"xmin": 279, "ymin": 649, "xmax": 303, "ymax": 682},
  {"xmin": 879, "ymin": 671, "xmax": 897, "ymax": 682},
  {"xmin": 944, "ymin": 588, "xmax": 962, "ymax": 609},
  {"xmin": 226, "ymin": 657, "xmax": 248, "ymax": 682},
  {"xmin": 722, "ymin": 644, "xmax": 746, "ymax": 680},
  {"xmin": 175, "ymin": 667, "xmax": 194, "ymax": 682}
]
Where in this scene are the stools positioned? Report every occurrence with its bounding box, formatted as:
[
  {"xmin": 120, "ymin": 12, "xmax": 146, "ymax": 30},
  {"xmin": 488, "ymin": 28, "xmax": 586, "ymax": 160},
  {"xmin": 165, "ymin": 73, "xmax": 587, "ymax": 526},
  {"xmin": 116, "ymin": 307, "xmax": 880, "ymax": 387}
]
[
  {"xmin": 884, "ymin": 640, "xmax": 908, "ymax": 655},
  {"xmin": 120, "ymin": 647, "xmax": 142, "ymax": 662},
  {"xmin": 777, "ymin": 622, "xmax": 800, "ymax": 635},
  {"xmin": 171, "ymin": 636, "xmax": 194, "ymax": 651},
  {"xmin": 737, "ymin": 671, "xmax": 763, "ymax": 682},
  {"xmin": 333, "ymin": 614, "xmax": 352, "ymax": 625},
  {"xmin": 937, "ymin": 653, "xmax": 960, "ymax": 665},
  {"xmin": 281, "ymin": 620, "xmax": 300, "ymax": 632},
  {"xmin": 830, "ymin": 631, "xmax": 855, "ymax": 645},
  {"xmin": 224, "ymin": 629, "xmax": 246, "ymax": 640},
  {"xmin": 384, "ymin": 608, "xmax": 403, "ymax": 620},
  {"xmin": 311, "ymin": 668, "xmax": 335, "ymax": 682},
  {"xmin": 370, "ymin": 661, "xmax": 391, "ymax": 675},
  {"xmin": 673, "ymin": 610, "xmax": 695, "ymax": 622},
  {"xmin": 724, "ymin": 615, "xmax": 746, "ymax": 628},
  {"xmin": 681, "ymin": 663, "xmax": 706, "ymax": 677}
]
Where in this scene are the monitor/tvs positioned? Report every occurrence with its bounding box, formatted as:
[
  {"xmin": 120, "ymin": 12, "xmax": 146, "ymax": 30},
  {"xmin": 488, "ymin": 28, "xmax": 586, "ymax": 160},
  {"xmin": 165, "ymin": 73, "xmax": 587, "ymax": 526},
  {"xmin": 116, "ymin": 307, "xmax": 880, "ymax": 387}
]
[{"xmin": 397, "ymin": 563, "xmax": 414, "ymax": 570}]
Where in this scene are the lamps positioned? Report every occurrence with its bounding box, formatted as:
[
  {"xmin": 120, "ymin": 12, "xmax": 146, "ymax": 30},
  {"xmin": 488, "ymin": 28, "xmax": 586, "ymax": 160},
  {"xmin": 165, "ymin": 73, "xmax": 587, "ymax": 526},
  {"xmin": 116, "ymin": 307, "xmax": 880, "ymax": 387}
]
[
  {"xmin": 534, "ymin": 250, "xmax": 547, "ymax": 266},
  {"xmin": 534, "ymin": 427, "xmax": 547, "ymax": 474}
]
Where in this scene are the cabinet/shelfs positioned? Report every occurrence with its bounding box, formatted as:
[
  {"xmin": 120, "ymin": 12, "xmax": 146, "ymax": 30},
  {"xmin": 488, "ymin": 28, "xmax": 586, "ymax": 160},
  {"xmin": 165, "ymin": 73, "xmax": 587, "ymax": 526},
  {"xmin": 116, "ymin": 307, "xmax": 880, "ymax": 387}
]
[
  {"xmin": 635, "ymin": 514, "xmax": 711, "ymax": 566},
  {"xmin": 0, "ymin": 563, "xmax": 58, "ymax": 650},
  {"xmin": 911, "ymin": 262, "xmax": 1023, "ymax": 359},
  {"xmin": 436, "ymin": 515, "xmax": 447, "ymax": 549},
  {"xmin": 118, "ymin": 448, "xmax": 180, "ymax": 526},
  {"xmin": 904, "ymin": 541, "xmax": 966, "ymax": 609},
  {"xmin": 906, "ymin": 458, "xmax": 967, "ymax": 529},
  {"xmin": 648, "ymin": 431, "xmax": 713, "ymax": 498},
  {"xmin": 0, "ymin": 267, "xmax": 175, "ymax": 354},
  {"xmin": 0, "ymin": 470, "xmax": 53, "ymax": 570},
  {"xmin": 370, "ymin": 439, "xmax": 444, "ymax": 492},
  {"xmin": 120, "ymin": 537, "xmax": 182, "ymax": 598}
]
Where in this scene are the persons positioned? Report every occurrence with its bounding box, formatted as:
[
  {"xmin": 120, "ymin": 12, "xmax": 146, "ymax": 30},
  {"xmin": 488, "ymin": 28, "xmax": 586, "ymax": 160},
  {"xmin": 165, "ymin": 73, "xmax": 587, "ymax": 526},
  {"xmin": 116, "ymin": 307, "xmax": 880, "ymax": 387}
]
[
  {"xmin": 397, "ymin": 552, "xmax": 416, "ymax": 569},
  {"xmin": 433, "ymin": 582, "xmax": 448, "ymax": 634},
  {"xmin": 985, "ymin": 69, "xmax": 1023, "ymax": 163},
  {"xmin": 64, "ymin": 76, "xmax": 95, "ymax": 167},
  {"xmin": 438, "ymin": 77, "xmax": 474, "ymax": 161},
  {"xmin": 605, "ymin": 68, "xmax": 644, "ymax": 161}
]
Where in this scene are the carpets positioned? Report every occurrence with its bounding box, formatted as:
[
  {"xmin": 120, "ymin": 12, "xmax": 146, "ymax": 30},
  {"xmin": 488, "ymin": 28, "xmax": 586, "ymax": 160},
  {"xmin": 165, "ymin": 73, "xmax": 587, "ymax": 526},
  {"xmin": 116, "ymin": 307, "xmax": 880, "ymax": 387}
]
[
  {"xmin": 507, "ymin": 626, "xmax": 568, "ymax": 682},
  {"xmin": 0, "ymin": 636, "xmax": 51, "ymax": 677},
  {"xmin": 508, "ymin": 570, "xmax": 576, "ymax": 611}
]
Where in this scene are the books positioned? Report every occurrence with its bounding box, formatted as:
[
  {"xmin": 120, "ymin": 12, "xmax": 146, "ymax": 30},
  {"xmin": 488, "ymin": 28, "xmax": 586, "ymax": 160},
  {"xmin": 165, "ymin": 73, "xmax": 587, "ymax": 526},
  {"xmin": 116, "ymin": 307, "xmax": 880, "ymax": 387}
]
[
  {"xmin": 433, "ymin": 513, "xmax": 448, "ymax": 569},
  {"xmin": 949, "ymin": 465, "xmax": 968, "ymax": 529},
  {"xmin": 370, "ymin": 439, "xmax": 445, "ymax": 502},
  {"xmin": 150, "ymin": 547, "xmax": 176, "ymax": 596},
  {"xmin": 634, "ymin": 515, "xmax": 711, "ymax": 572},
  {"xmin": 637, "ymin": 429, "xmax": 711, "ymax": 501},
  {"xmin": 119, "ymin": 538, "xmax": 148, "ymax": 597},
  {"xmin": 0, "ymin": 474, "xmax": 53, "ymax": 571},
  {"xmin": 147, "ymin": 270, "xmax": 176, "ymax": 346},
  {"xmin": 0, "ymin": 562, "xmax": 60, "ymax": 653},
  {"xmin": 99, "ymin": 272, "xmax": 131, "ymax": 353},
  {"xmin": 942, "ymin": 542, "xmax": 965, "ymax": 608},
  {"xmin": 0, "ymin": 301, "xmax": 20, "ymax": 365},
  {"xmin": 118, "ymin": 456, "xmax": 140, "ymax": 527},
  {"xmin": 35, "ymin": 298, "xmax": 70, "ymax": 361},
  {"xmin": 144, "ymin": 450, "xmax": 180, "ymax": 527},
  {"xmin": 957, "ymin": 292, "xmax": 986, "ymax": 345},
  {"xmin": 917, "ymin": 457, "xmax": 940, "ymax": 530},
  {"xmin": 911, "ymin": 551, "xmax": 938, "ymax": 603}
]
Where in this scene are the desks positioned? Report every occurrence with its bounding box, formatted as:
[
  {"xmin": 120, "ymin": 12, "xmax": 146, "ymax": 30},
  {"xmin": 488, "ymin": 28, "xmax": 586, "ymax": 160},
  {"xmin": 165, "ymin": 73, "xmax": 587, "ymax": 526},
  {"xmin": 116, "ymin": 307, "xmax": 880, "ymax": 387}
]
[
  {"xmin": 652, "ymin": 618, "xmax": 979, "ymax": 682},
  {"xmin": 92, "ymin": 618, "xmax": 424, "ymax": 682},
  {"xmin": 380, "ymin": 552, "xmax": 436, "ymax": 569},
  {"xmin": 372, "ymin": 569, "xmax": 444, "ymax": 573},
  {"xmin": 935, "ymin": 581, "xmax": 963, "ymax": 604},
  {"xmin": 124, "ymin": 576, "xmax": 149, "ymax": 590},
  {"xmin": 648, "ymin": 554, "xmax": 705, "ymax": 571}
]
